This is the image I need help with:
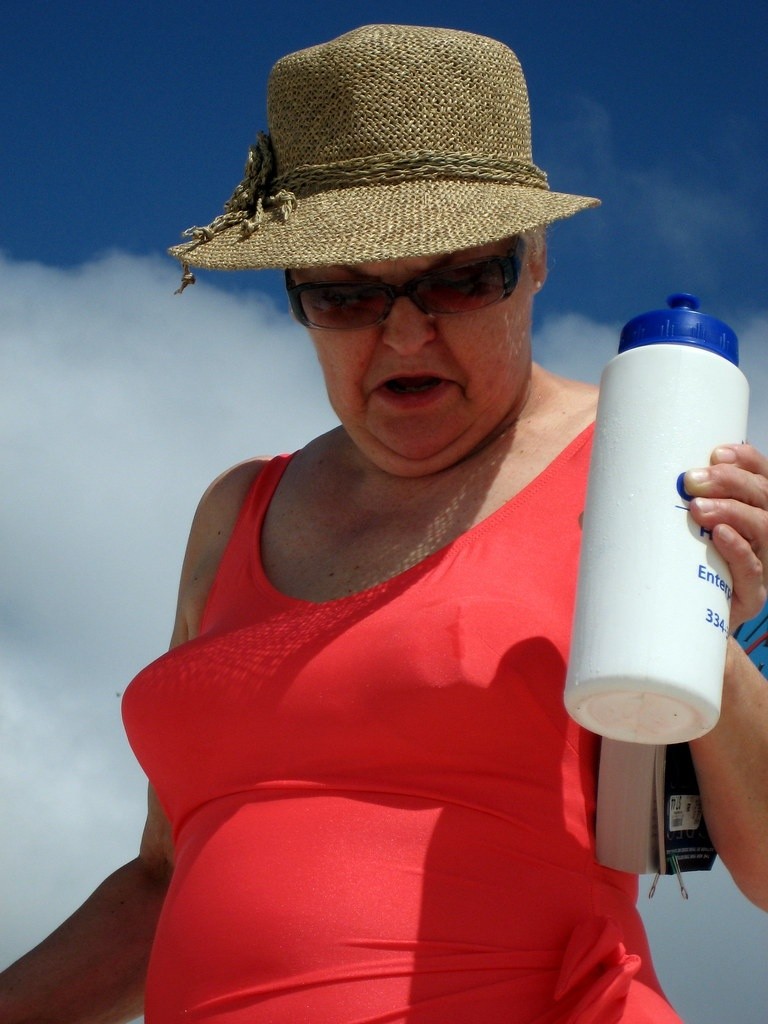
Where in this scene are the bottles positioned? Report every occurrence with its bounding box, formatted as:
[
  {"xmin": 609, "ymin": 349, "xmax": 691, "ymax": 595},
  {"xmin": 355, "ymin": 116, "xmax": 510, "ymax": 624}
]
[{"xmin": 564, "ymin": 293, "xmax": 749, "ymax": 746}]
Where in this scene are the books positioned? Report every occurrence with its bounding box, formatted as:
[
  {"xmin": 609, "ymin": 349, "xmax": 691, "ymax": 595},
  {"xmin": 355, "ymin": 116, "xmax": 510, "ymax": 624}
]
[{"xmin": 594, "ymin": 738, "xmax": 718, "ymax": 875}]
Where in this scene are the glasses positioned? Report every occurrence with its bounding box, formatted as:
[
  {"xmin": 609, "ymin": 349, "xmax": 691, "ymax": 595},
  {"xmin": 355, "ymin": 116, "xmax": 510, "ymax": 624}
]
[{"xmin": 285, "ymin": 234, "xmax": 527, "ymax": 331}]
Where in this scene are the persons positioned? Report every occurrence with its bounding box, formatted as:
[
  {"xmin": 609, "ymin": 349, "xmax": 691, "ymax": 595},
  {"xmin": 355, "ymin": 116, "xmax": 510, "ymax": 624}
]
[{"xmin": 0, "ymin": 25, "xmax": 768, "ymax": 1024}]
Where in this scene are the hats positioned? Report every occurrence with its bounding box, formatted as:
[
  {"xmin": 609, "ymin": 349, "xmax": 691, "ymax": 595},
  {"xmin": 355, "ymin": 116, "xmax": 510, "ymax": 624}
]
[{"xmin": 168, "ymin": 21, "xmax": 600, "ymax": 270}]
